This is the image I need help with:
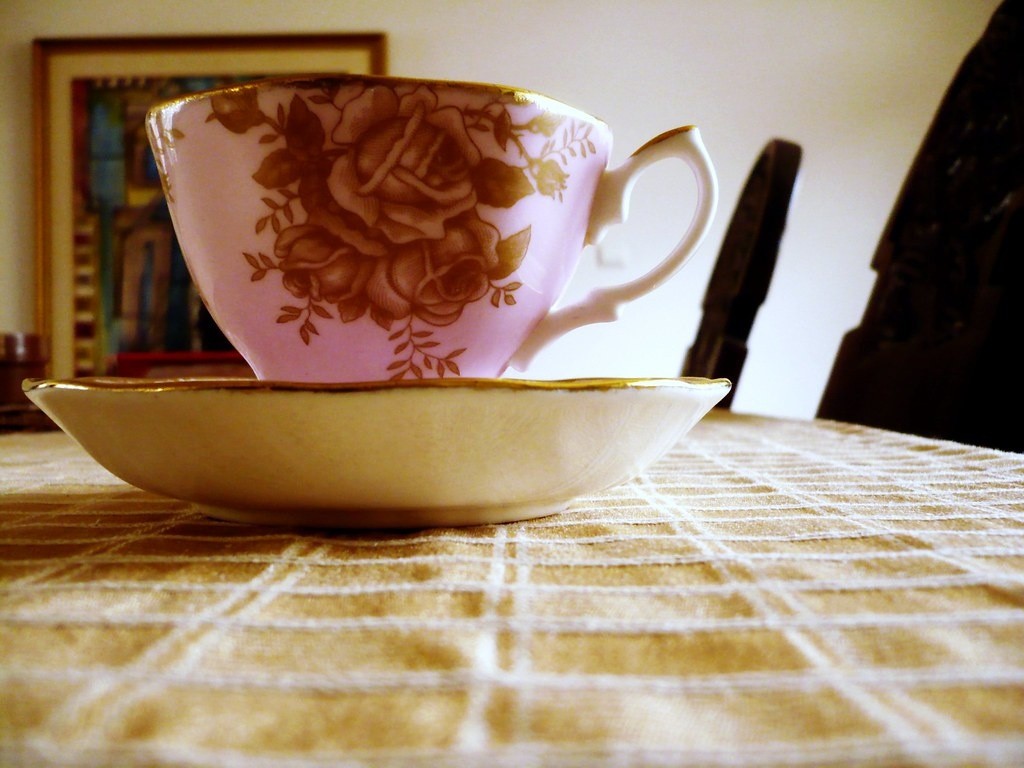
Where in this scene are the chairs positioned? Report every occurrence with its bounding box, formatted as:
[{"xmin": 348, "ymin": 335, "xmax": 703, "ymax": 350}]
[
  {"xmin": 681, "ymin": 140, "xmax": 803, "ymax": 413},
  {"xmin": 815, "ymin": 0, "xmax": 1023, "ymax": 454}
]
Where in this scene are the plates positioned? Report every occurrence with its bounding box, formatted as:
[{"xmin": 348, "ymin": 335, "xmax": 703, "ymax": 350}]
[{"xmin": 20, "ymin": 374, "xmax": 731, "ymax": 529}]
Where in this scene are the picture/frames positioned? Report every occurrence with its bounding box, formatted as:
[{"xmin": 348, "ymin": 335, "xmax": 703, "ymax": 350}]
[{"xmin": 31, "ymin": 31, "xmax": 388, "ymax": 379}]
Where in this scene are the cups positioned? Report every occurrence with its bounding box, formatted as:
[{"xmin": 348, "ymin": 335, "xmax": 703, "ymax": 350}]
[{"xmin": 146, "ymin": 73, "xmax": 720, "ymax": 385}]
